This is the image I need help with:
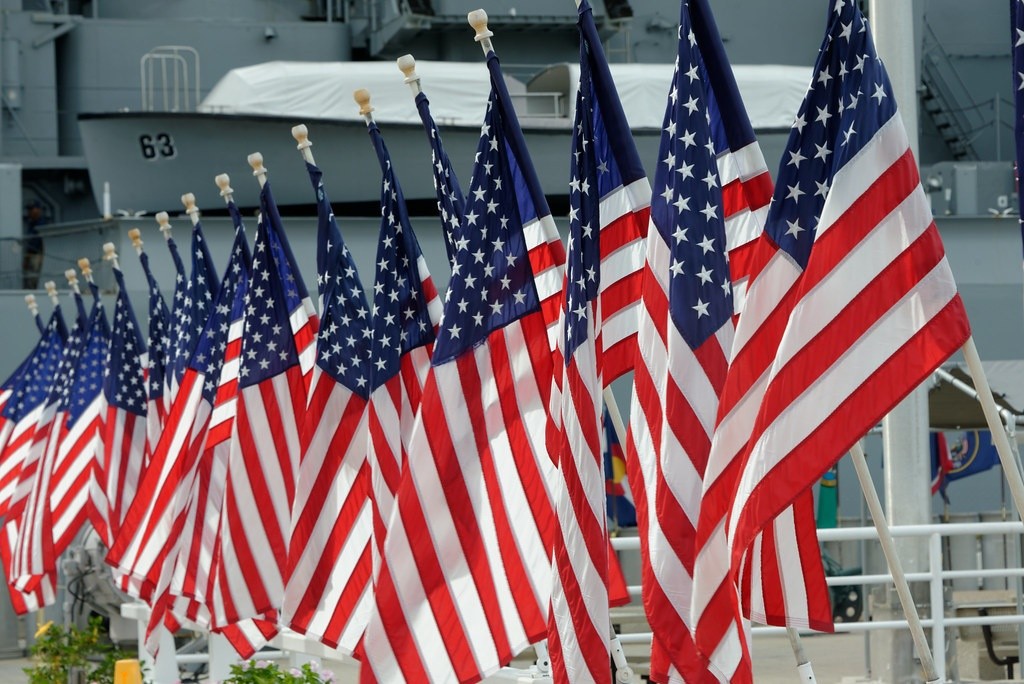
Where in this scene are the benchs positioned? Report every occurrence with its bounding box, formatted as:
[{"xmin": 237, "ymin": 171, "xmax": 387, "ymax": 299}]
[{"xmin": 956, "ymin": 601, "xmax": 1021, "ymax": 680}]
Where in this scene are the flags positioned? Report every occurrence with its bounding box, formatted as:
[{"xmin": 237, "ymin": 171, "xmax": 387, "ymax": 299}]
[
  {"xmin": 630, "ymin": 0, "xmax": 834, "ymax": 684},
  {"xmin": 371, "ymin": 128, "xmax": 444, "ymax": 589},
  {"xmin": 205, "ymin": 179, "xmax": 319, "ymax": 660},
  {"xmin": 0, "ymin": 172, "xmax": 250, "ymax": 656},
  {"xmin": 547, "ymin": 2, "xmax": 652, "ymax": 684},
  {"xmin": 359, "ymin": 50, "xmax": 555, "ymax": 683},
  {"xmin": 690, "ymin": 0, "xmax": 970, "ymax": 684},
  {"xmin": 416, "ymin": 93, "xmax": 465, "ymax": 262},
  {"xmin": 279, "ymin": 161, "xmax": 373, "ymax": 658}
]
